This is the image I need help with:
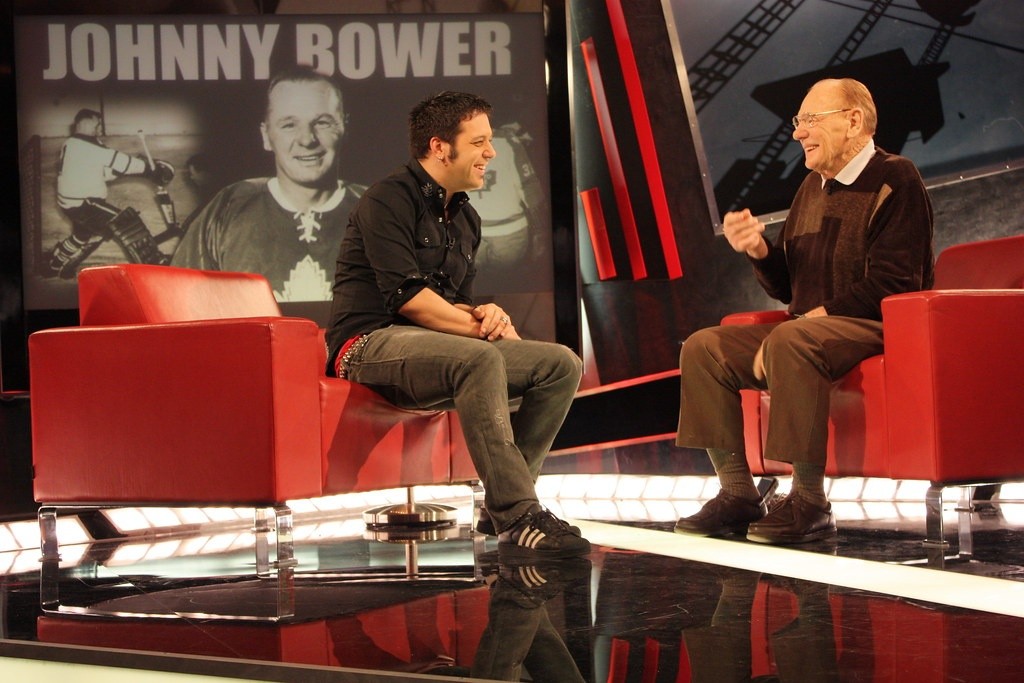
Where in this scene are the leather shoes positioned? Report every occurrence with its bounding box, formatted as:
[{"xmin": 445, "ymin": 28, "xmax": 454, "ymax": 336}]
[
  {"xmin": 674, "ymin": 489, "xmax": 769, "ymax": 537},
  {"xmin": 747, "ymin": 495, "xmax": 837, "ymax": 543}
]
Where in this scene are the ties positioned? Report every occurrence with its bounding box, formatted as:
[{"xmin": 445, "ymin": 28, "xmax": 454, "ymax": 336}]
[{"xmin": 825, "ymin": 178, "xmax": 838, "ymax": 194}]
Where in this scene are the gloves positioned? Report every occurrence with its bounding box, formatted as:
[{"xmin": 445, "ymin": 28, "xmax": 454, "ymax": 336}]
[{"xmin": 142, "ymin": 158, "xmax": 174, "ymax": 185}]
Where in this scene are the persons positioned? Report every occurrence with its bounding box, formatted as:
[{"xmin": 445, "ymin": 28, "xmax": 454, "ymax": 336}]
[
  {"xmin": 181, "ymin": 153, "xmax": 243, "ymax": 236},
  {"xmin": 682, "ymin": 565, "xmax": 840, "ymax": 683},
  {"xmin": 168, "ymin": 66, "xmax": 369, "ymax": 303},
  {"xmin": 674, "ymin": 78, "xmax": 935, "ymax": 545},
  {"xmin": 324, "ymin": 89, "xmax": 593, "ymax": 560},
  {"xmin": 48, "ymin": 108, "xmax": 175, "ymax": 280},
  {"xmin": 467, "ymin": 125, "xmax": 547, "ymax": 292},
  {"xmin": 471, "ymin": 559, "xmax": 589, "ymax": 683}
]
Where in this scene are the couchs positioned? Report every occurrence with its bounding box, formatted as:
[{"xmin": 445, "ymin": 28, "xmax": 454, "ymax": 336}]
[
  {"xmin": 719, "ymin": 235, "xmax": 1024, "ymax": 549},
  {"xmin": 27, "ymin": 262, "xmax": 480, "ymax": 569}
]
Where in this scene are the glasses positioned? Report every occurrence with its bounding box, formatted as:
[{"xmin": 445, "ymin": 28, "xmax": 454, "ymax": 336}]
[{"xmin": 792, "ymin": 109, "xmax": 852, "ymax": 130}]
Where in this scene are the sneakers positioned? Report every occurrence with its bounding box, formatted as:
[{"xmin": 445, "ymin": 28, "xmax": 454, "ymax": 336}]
[
  {"xmin": 477, "ymin": 550, "xmax": 499, "ymax": 576},
  {"xmin": 499, "ymin": 558, "xmax": 592, "ymax": 599},
  {"xmin": 498, "ymin": 509, "xmax": 591, "ymax": 559},
  {"xmin": 476, "ymin": 508, "xmax": 581, "ymax": 538}
]
